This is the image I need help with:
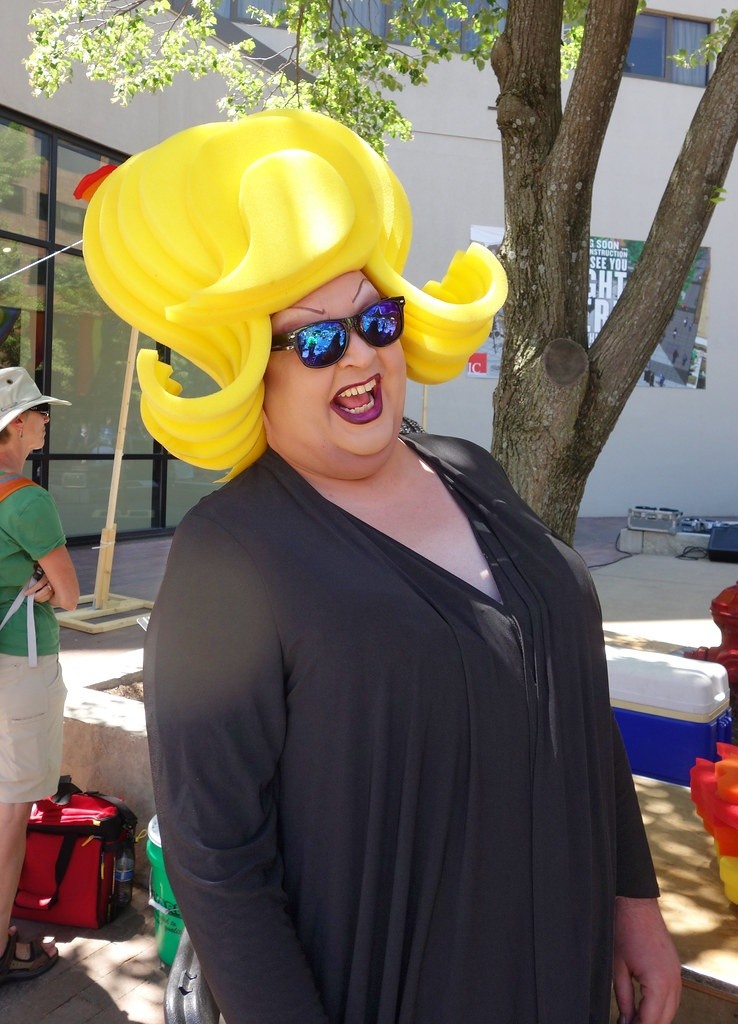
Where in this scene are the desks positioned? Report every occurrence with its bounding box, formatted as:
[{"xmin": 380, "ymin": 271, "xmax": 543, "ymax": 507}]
[{"xmin": 609, "ymin": 773, "xmax": 738, "ymax": 1024}]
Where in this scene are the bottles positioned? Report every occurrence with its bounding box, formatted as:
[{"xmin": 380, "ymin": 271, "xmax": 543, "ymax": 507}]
[{"xmin": 114, "ymin": 841, "xmax": 134, "ymax": 909}]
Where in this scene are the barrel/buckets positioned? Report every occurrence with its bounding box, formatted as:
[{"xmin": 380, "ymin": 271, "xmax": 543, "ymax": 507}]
[{"xmin": 147, "ymin": 813, "xmax": 185, "ymax": 966}]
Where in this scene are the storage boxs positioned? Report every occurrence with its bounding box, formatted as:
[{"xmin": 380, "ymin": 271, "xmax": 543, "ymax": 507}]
[{"xmin": 605, "ymin": 645, "xmax": 731, "ymax": 788}]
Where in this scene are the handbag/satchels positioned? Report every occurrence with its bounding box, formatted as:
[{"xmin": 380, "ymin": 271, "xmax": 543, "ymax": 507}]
[{"xmin": 11, "ymin": 775, "xmax": 137, "ymax": 929}]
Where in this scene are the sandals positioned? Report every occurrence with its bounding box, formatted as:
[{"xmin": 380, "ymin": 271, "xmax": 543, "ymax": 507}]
[{"xmin": 0, "ymin": 937, "xmax": 59, "ymax": 980}]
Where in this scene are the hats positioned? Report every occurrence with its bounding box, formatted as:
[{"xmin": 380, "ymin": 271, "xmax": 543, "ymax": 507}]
[{"xmin": 0, "ymin": 367, "xmax": 73, "ymax": 432}]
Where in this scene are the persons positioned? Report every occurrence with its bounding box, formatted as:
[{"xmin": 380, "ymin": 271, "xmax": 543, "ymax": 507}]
[
  {"xmin": 75, "ymin": 111, "xmax": 683, "ymax": 1024},
  {"xmin": 0, "ymin": 367, "xmax": 80, "ymax": 982}
]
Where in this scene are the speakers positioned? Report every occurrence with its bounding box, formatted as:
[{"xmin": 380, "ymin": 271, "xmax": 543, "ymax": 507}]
[{"xmin": 707, "ymin": 526, "xmax": 738, "ymax": 564}]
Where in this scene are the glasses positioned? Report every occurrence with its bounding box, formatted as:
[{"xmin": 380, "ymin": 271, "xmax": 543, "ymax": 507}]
[
  {"xmin": 28, "ymin": 402, "xmax": 49, "ymax": 417},
  {"xmin": 272, "ymin": 296, "xmax": 405, "ymax": 369}
]
